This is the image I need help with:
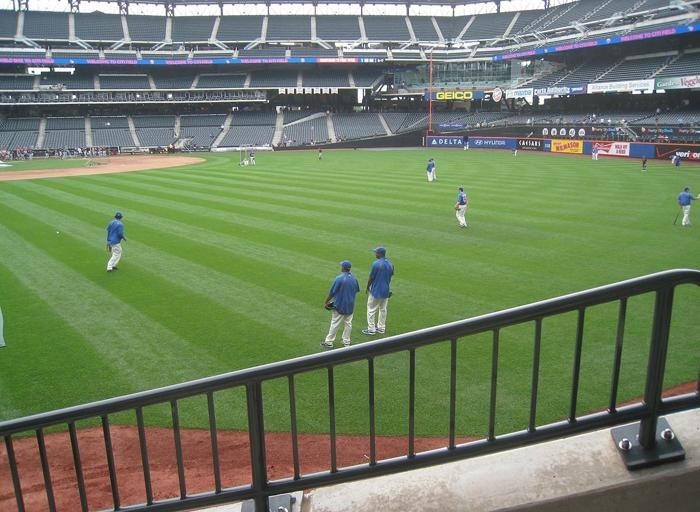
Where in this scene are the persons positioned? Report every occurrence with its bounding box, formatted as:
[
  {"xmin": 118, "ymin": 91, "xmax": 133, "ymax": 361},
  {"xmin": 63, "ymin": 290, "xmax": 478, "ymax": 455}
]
[
  {"xmin": 677, "ymin": 186, "xmax": 700, "ymax": 228},
  {"xmin": 430, "ymin": 158, "xmax": 436, "ymax": 180},
  {"xmin": 591, "ymin": 143, "xmax": 598, "ymax": 160},
  {"xmin": 238, "ymin": 138, "xmax": 324, "ymax": 168},
  {"xmin": 0, "ymin": 89, "xmax": 175, "ymax": 159},
  {"xmin": 104, "ymin": 211, "xmax": 128, "ymax": 274},
  {"xmin": 1, "ymin": 310, "xmax": 6, "ymax": 353},
  {"xmin": 453, "ymin": 187, "xmax": 470, "ymax": 228},
  {"xmin": 319, "ymin": 259, "xmax": 360, "ymax": 350},
  {"xmin": 671, "ymin": 154, "xmax": 681, "ymax": 171},
  {"xmin": 512, "ymin": 147, "xmax": 517, "ymax": 158},
  {"xmin": 465, "ymin": 103, "xmax": 700, "ymax": 129},
  {"xmin": 641, "ymin": 156, "xmax": 648, "ymax": 172},
  {"xmin": 361, "ymin": 246, "xmax": 394, "ymax": 336},
  {"xmin": 427, "ymin": 158, "xmax": 435, "ymax": 182}
]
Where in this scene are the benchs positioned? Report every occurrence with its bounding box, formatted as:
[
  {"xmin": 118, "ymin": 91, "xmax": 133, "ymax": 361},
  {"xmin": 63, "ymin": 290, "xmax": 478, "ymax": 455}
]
[{"xmin": 0, "ymin": 1, "xmax": 699, "ymax": 153}]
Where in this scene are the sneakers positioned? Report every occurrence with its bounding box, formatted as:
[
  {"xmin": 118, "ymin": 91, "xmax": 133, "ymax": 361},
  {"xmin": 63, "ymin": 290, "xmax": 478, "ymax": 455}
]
[
  {"xmin": 321, "ymin": 342, "xmax": 333, "ymax": 347},
  {"xmin": 362, "ymin": 328, "xmax": 385, "ymax": 335}
]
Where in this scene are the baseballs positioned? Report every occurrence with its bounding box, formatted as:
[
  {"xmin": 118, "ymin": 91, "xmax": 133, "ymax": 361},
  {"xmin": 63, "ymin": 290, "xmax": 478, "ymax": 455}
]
[{"xmin": 57, "ymin": 231, "xmax": 59, "ymax": 234}]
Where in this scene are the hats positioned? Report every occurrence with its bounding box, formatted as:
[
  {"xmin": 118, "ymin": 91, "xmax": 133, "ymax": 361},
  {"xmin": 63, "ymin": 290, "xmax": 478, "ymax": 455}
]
[
  {"xmin": 340, "ymin": 261, "xmax": 351, "ymax": 269},
  {"xmin": 374, "ymin": 247, "xmax": 386, "ymax": 254}
]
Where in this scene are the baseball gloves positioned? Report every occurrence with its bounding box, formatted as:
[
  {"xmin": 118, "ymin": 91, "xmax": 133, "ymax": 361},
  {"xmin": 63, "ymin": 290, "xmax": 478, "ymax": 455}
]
[{"xmin": 325, "ymin": 302, "xmax": 336, "ymax": 310}]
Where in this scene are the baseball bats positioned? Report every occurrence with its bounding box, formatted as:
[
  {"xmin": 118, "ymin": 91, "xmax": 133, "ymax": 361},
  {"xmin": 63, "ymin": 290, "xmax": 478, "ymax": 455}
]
[{"xmin": 673, "ymin": 207, "xmax": 682, "ymax": 224}]
